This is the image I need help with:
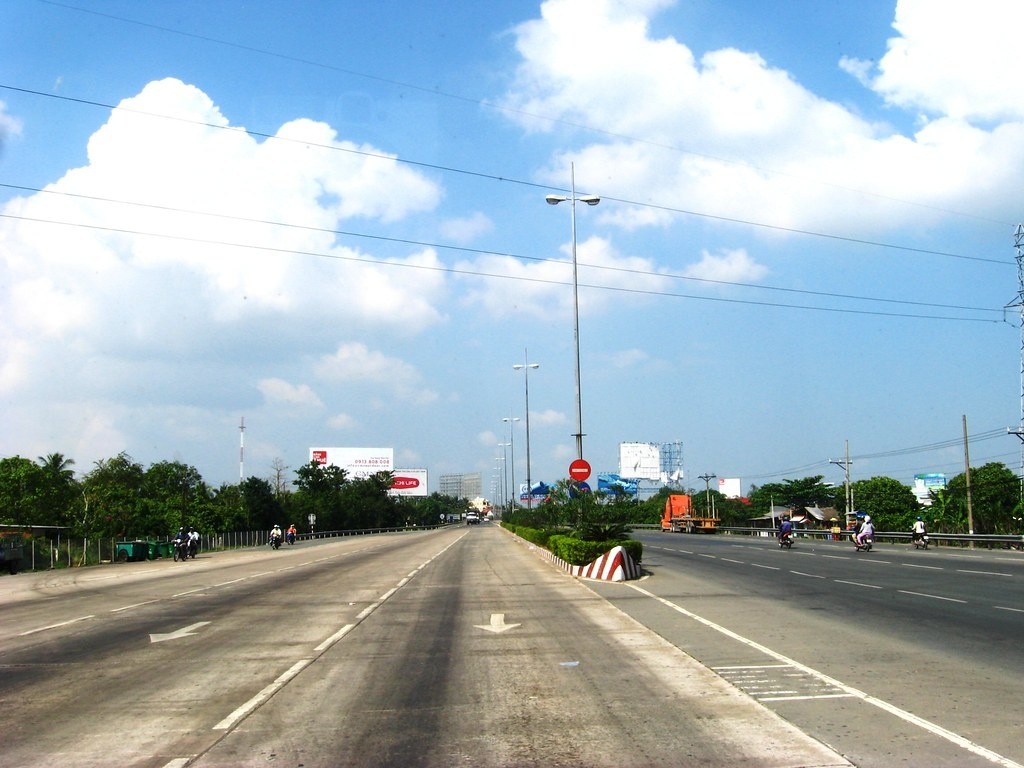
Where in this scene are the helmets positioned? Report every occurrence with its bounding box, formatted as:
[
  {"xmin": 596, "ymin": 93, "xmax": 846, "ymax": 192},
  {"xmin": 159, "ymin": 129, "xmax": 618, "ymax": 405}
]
[
  {"xmin": 274, "ymin": 525, "xmax": 278, "ymax": 528},
  {"xmin": 783, "ymin": 516, "xmax": 788, "ymax": 520},
  {"xmin": 180, "ymin": 526, "xmax": 183, "ymax": 530},
  {"xmin": 865, "ymin": 516, "xmax": 871, "ymax": 522},
  {"xmin": 291, "ymin": 524, "xmax": 294, "ymax": 527},
  {"xmin": 190, "ymin": 526, "xmax": 193, "ymax": 530},
  {"xmin": 917, "ymin": 516, "xmax": 922, "ymax": 521}
]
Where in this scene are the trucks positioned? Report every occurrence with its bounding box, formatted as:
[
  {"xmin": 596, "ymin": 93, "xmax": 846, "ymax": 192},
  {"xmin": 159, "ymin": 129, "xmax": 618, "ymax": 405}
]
[{"xmin": 466, "ymin": 512, "xmax": 480, "ymax": 525}]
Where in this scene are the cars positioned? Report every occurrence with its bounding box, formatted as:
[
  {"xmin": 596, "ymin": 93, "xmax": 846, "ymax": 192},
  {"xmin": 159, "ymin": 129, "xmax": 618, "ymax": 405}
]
[{"xmin": 483, "ymin": 516, "xmax": 489, "ymax": 522}]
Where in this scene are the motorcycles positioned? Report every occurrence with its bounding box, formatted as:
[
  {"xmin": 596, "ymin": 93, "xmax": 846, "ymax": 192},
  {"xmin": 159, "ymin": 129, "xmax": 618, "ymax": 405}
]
[
  {"xmin": 287, "ymin": 532, "xmax": 296, "ymax": 545},
  {"xmin": 270, "ymin": 534, "xmax": 281, "ymax": 551}
]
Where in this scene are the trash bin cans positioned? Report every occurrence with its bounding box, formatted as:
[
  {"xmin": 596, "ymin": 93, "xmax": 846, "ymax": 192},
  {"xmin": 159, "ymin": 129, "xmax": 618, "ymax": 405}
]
[
  {"xmin": 146, "ymin": 540, "xmax": 175, "ymax": 560},
  {"xmin": 115, "ymin": 541, "xmax": 147, "ymax": 562}
]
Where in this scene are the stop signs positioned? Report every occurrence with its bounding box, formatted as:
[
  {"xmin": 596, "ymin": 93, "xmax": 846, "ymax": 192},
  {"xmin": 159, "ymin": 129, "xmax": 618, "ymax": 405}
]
[{"xmin": 569, "ymin": 459, "xmax": 592, "ymax": 481}]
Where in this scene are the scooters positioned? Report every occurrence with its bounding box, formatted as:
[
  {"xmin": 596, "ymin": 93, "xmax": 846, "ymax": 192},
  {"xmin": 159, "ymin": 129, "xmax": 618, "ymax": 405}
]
[
  {"xmin": 909, "ymin": 526, "xmax": 931, "ymax": 550},
  {"xmin": 852, "ymin": 524, "xmax": 873, "ymax": 552},
  {"xmin": 777, "ymin": 525, "xmax": 794, "ymax": 549},
  {"xmin": 172, "ymin": 537, "xmax": 197, "ymax": 562}
]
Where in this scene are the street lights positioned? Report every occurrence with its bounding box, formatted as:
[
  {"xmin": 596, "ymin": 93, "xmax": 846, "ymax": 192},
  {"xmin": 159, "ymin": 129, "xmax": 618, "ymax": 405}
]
[
  {"xmin": 545, "ymin": 162, "xmax": 600, "ymax": 528},
  {"xmin": 502, "ymin": 411, "xmax": 520, "ymax": 515},
  {"xmin": 490, "ymin": 455, "xmax": 505, "ymax": 520},
  {"xmin": 512, "ymin": 348, "xmax": 540, "ymax": 515},
  {"xmin": 498, "ymin": 435, "xmax": 512, "ymax": 514}
]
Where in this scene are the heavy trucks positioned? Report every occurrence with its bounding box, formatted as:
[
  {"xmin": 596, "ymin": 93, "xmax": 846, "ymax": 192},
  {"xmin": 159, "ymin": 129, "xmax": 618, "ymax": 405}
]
[{"xmin": 660, "ymin": 495, "xmax": 721, "ymax": 534}]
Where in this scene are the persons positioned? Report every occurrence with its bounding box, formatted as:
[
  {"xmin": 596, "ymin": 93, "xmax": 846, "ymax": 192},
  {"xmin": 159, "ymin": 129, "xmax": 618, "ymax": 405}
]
[
  {"xmin": 187, "ymin": 526, "xmax": 201, "ymax": 551},
  {"xmin": 911, "ymin": 516, "xmax": 927, "ymax": 545},
  {"xmin": 286, "ymin": 525, "xmax": 297, "ymax": 541},
  {"xmin": 172, "ymin": 526, "xmax": 191, "ymax": 557},
  {"xmin": 269, "ymin": 525, "xmax": 282, "ymax": 546},
  {"xmin": 851, "ymin": 515, "xmax": 874, "ymax": 544},
  {"xmin": 777, "ymin": 515, "xmax": 793, "ymax": 542}
]
